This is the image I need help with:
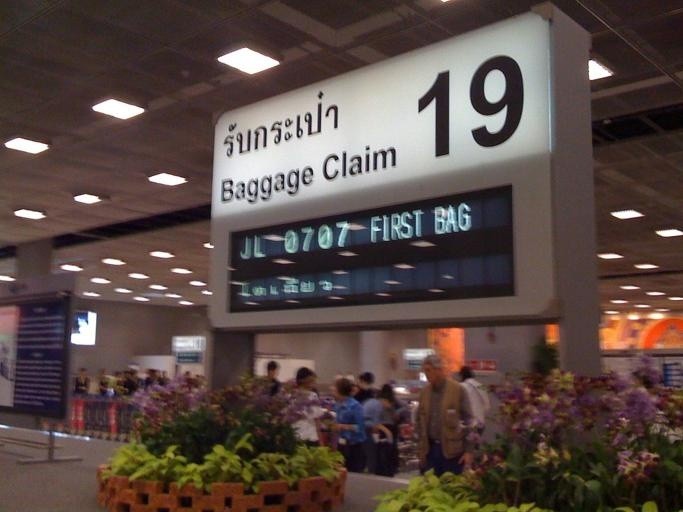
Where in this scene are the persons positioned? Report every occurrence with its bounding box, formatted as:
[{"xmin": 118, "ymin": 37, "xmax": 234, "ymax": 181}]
[
  {"xmin": 631, "ymin": 370, "xmax": 647, "ymax": 392},
  {"xmin": 74, "ymin": 365, "xmax": 205, "ymax": 400},
  {"xmin": 254, "ymin": 355, "xmax": 492, "ymax": 478}
]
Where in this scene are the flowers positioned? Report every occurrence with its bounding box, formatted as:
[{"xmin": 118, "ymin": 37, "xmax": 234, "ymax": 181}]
[
  {"xmin": 99, "ymin": 377, "xmax": 342, "ymax": 491},
  {"xmin": 372, "ymin": 348, "xmax": 683, "ymax": 512}
]
